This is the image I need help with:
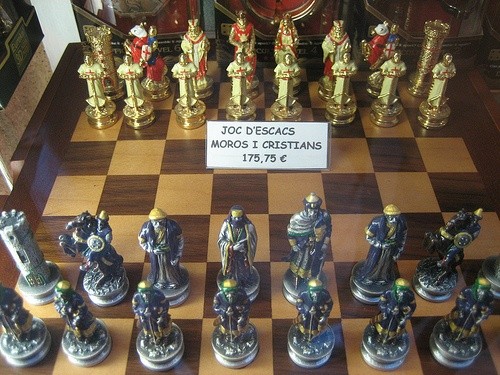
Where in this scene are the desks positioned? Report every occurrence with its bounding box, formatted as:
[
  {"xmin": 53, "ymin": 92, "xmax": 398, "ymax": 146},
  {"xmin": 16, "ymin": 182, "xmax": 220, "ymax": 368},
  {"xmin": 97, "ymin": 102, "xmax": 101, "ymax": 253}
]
[{"xmin": 0, "ymin": 42, "xmax": 500, "ymax": 375}]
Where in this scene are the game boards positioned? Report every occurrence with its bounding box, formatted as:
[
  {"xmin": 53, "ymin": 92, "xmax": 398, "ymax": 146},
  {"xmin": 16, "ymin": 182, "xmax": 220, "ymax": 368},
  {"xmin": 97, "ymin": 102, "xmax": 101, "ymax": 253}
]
[{"xmin": 0, "ymin": 38, "xmax": 500, "ymax": 375}]
